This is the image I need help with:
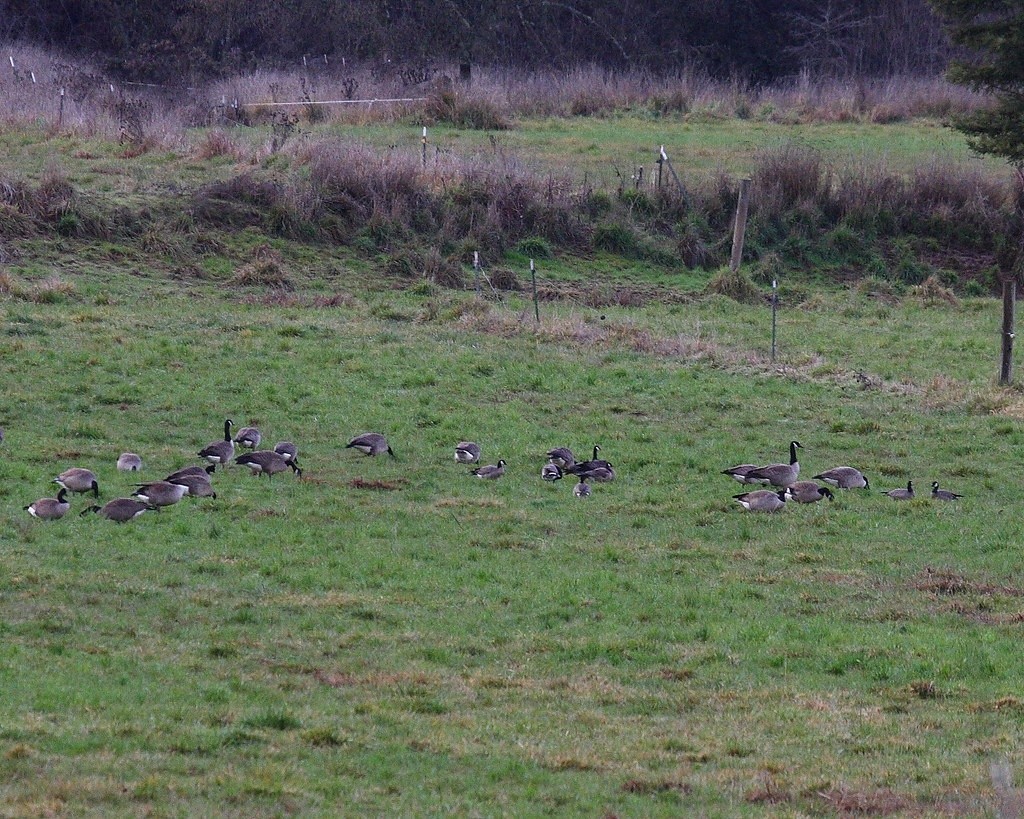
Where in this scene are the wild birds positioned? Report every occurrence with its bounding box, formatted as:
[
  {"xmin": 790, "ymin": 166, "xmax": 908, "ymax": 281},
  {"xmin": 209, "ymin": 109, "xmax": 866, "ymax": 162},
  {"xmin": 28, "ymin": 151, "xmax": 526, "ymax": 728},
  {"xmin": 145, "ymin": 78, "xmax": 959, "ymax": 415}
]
[
  {"xmin": 811, "ymin": 466, "xmax": 872, "ymax": 490},
  {"xmin": 345, "ymin": 431, "xmax": 615, "ymax": 483},
  {"xmin": 572, "ymin": 476, "xmax": 591, "ymax": 496},
  {"xmin": 162, "ymin": 465, "xmax": 217, "ymax": 483},
  {"xmin": 78, "ymin": 499, "xmax": 158, "ymax": 522},
  {"xmin": 116, "ymin": 453, "xmax": 141, "ymax": 472},
  {"xmin": 169, "ymin": 476, "xmax": 217, "ymax": 500},
  {"xmin": 720, "ymin": 464, "xmax": 770, "ymax": 488},
  {"xmin": 196, "ymin": 419, "xmax": 236, "ymax": 470},
  {"xmin": 778, "ymin": 481, "xmax": 834, "ymax": 503},
  {"xmin": 930, "ymin": 481, "xmax": 965, "ymax": 500},
  {"xmin": 732, "ymin": 490, "xmax": 793, "ymax": 512},
  {"xmin": 131, "ymin": 480, "xmax": 197, "ymax": 513},
  {"xmin": 234, "ymin": 450, "xmax": 303, "ymax": 484},
  {"xmin": 274, "ymin": 442, "xmax": 299, "ymax": 464},
  {"xmin": 231, "ymin": 426, "xmax": 261, "ymax": 453},
  {"xmin": 21, "ymin": 487, "xmax": 72, "ymax": 521},
  {"xmin": 880, "ymin": 480, "xmax": 916, "ymax": 499},
  {"xmin": 747, "ymin": 441, "xmax": 805, "ymax": 487},
  {"xmin": 49, "ymin": 470, "xmax": 99, "ymax": 499}
]
